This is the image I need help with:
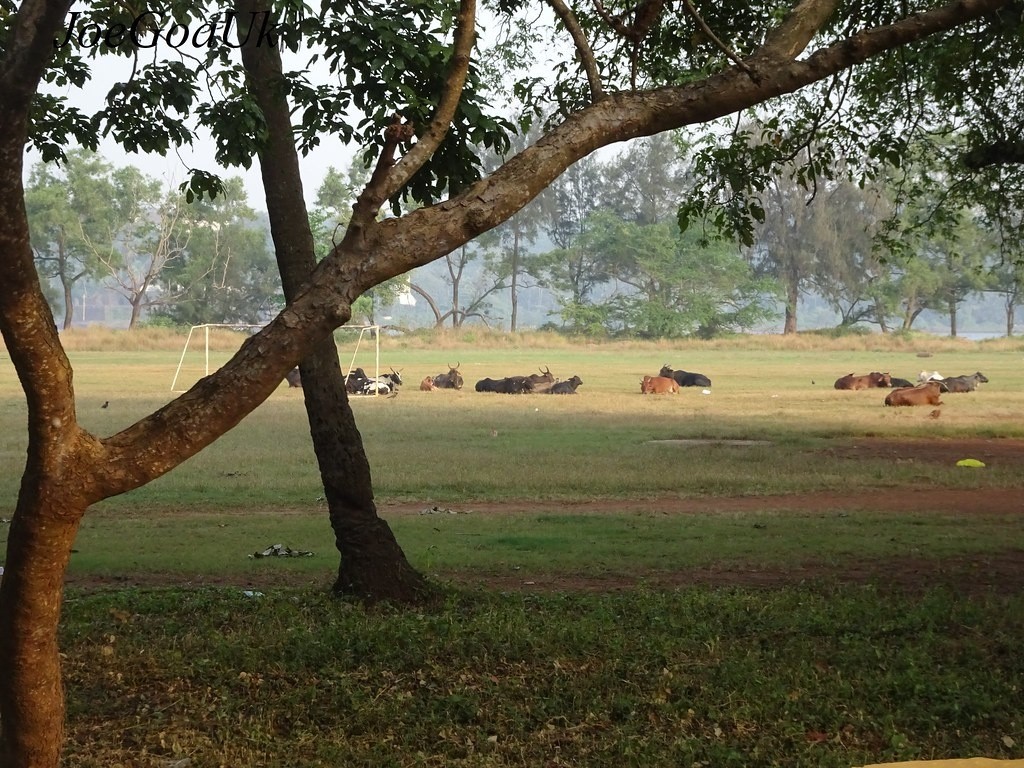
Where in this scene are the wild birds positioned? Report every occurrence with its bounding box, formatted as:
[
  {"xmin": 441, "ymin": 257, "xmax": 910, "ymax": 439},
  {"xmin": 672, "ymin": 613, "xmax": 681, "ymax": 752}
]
[{"xmin": 101, "ymin": 400, "xmax": 109, "ymax": 409}]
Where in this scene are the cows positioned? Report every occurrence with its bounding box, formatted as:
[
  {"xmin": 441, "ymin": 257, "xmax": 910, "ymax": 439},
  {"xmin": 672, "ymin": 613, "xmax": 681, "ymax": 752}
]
[{"xmin": 281, "ymin": 362, "xmax": 990, "ymax": 407}]
